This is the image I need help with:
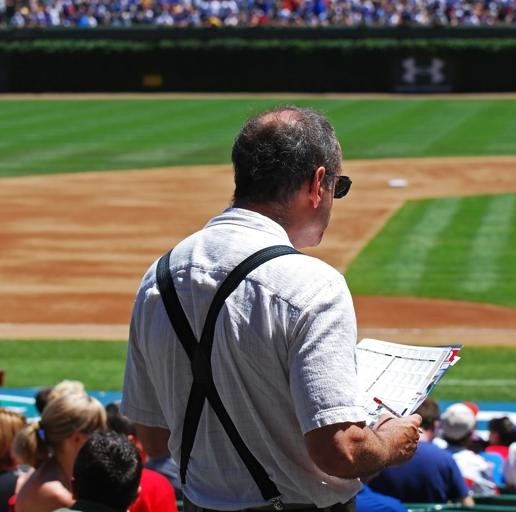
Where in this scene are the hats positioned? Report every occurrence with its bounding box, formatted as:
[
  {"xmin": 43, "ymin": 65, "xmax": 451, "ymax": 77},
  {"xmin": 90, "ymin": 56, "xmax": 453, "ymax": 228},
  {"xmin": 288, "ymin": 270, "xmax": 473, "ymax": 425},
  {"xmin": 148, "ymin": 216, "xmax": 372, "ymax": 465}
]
[{"xmin": 439, "ymin": 401, "xmax": 479, "ymax": 441}]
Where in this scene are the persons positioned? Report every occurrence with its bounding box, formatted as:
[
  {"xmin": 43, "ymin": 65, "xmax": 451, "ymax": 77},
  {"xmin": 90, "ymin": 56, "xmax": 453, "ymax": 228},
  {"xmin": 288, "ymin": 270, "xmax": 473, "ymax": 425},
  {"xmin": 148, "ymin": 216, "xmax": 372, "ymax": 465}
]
[
  {"xmin": 33, "ymin": 389, "xmax": 49, "ymax": 440},
  {"xmin": 142, "ymin": 423, "xmax": 182, "ymax": 491},
  {"xmin": 463, "ymin": 402, "xmax": 488, "ymax": 444},
  {"xmin": 368, "ymin": 397, "xmax": 475, "ymax": 508},
  {"xmin": 119, "ymin": 106, "xmax": 424, "ymax": 512},
  {"xmin": 486, "ymin": 417, "xmax": 516, "ymax": 461},
  {"xmin": 7, "ymin": 419, "xmax": 44, "ymax": 512},
  {"xmin": 104, "ymin": 402, "xmax": 138, "ymax": 436},
  {"xmin": 349, "ymin": 481, "xmax": 410, "ymax": 512},
  {"xmin": 439, "ymin": 403, "xmax": 500, "ymax": 495},
  {"xmin": 0, "ymin": 409, "xmax": 33, "ymax": 511},
  {"xmin": 119, "ymin": 429, "xmax": 178, "ymax": 512},
  {"xmin": 54, "ymin": 429, "xmax": 144, "ymax": 512},
  {"xmin": 14, "ymin": 395, "xmax": 108, "ymax": 512},
  {"xmin": 46, "ymin": 379, "xmax": 87, "ymax": 404}
]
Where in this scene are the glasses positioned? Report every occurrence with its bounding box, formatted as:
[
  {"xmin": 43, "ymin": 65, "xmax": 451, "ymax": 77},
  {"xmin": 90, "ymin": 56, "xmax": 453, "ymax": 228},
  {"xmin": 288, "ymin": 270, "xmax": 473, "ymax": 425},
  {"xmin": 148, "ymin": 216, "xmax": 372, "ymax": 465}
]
[{"xmin": 327, "ymin": 175, "xmax": 352, "ymax": 199}]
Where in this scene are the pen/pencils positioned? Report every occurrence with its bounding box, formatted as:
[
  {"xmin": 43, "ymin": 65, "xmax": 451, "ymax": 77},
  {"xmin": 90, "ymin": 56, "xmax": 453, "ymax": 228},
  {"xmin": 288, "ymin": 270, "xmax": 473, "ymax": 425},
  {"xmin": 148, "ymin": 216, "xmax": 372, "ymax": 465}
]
[{"xmin": 373, "ymin": 396, "xmax": 423, "ymax": 434}]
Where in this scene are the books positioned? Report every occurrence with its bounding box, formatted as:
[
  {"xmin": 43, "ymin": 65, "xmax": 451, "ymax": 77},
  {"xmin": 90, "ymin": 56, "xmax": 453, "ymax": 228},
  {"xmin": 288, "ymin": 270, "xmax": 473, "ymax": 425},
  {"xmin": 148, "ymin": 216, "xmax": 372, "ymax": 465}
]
[{"xmin": 355, "ymin": 337, "xmax": 465, "ymax": 428}]
